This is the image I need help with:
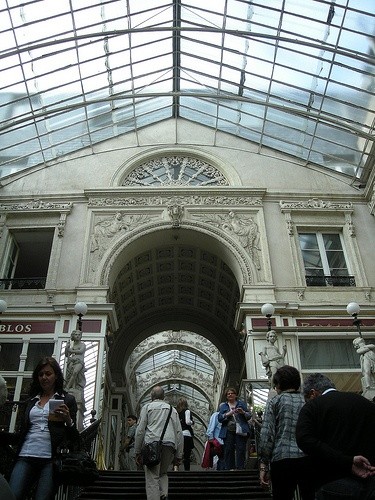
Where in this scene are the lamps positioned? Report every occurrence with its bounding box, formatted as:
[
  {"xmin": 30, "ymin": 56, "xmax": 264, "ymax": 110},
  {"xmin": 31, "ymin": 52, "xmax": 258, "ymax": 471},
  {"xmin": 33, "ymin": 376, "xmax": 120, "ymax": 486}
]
[
  {"xmin": 346, "ymin": 302, "xmax": 362, "ymax": 338},
  {"xmin": 261, "ymin": 303, "xmax": 275, "ymax": 332},
  {"xmin": 0, "ymin": 300, "xmax": 7, "ymax": 316},
  {"xmin": 73, "ymin": 302, "xmax": 88, "ymax": 332}
]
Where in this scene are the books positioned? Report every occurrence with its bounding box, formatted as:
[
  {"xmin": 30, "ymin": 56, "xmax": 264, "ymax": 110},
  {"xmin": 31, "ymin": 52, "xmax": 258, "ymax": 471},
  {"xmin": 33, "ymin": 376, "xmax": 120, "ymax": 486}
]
[{"xmin": 49, "ymin": 399, "xmax": 64, "ymax": 422}]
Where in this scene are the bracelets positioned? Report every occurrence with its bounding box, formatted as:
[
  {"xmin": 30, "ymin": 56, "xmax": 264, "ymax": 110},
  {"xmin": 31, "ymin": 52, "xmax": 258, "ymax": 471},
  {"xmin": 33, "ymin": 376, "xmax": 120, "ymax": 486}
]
[{"xmin": 260, "ymin": 467, "xmax": 269, "ymax": 471}]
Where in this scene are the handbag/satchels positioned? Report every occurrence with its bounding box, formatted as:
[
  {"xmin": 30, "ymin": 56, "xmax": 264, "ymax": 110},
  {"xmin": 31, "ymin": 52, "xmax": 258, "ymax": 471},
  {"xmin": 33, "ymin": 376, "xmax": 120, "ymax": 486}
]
[
  {"xmin": 53, "ymin": 435, "xmax": 99, "ymax": 486},
  {"xmin": 142, "ymin": 441, "xmax": 165, "ymax": 467},
  {"xmin": 235, "ymin": 422, "xmax": 247, "ymax": 436}
]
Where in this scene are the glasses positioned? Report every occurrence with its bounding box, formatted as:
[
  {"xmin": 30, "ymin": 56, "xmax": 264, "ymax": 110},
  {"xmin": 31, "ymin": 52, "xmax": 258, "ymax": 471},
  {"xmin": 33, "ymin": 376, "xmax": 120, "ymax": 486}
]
[{"xmin": 273, "ymin": 385, "xmax": 277, "ymax": 390}]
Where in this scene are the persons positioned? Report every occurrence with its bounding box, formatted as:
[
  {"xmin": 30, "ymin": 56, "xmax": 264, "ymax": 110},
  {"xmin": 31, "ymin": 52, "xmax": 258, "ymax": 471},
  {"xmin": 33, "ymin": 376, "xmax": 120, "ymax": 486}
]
[
  {"xmin": 201, "ymin": 412, "xmax": 224, "ymax": 470},
  {"xmin": 258, "ymin": 365, "xmax": 316, "ymax": 500},
  {"xmin": 119, "ymin": 415, "xmax": 143, "ymax": 470},
  {"xmin": 174, "ymin": 398, "xmax": 195, "ymax": 471},
  {"xmin": 134, "ymin": 386, "xmax": 184, "ymax": 500},
  {"xmin": 218, "ymin": 388, "xmax": 252, "ymax": 470},
  {"xmin": 9, "ymin": 356, "xmax": 78, "ymax": 500},
  {"xmin": 229, "ymin": 211, "xmax": 261, "ymax": 270},
  {"xmin": 261, "ymin": 329, "xmax": 287, "ymax": 384},
  {"xmin": 65, "ymin": 330, "xmax": 86, "ymax": 390},
  {"xmin": 91, "ymin": 212, "xmax": 122, "ymax": 253},
  {"xmin": 353, "ymin": 337, "xmax": 375, "ymax": 389},
  {"xmin": 296, "ymin": 372, "xmax": 375, "ymax": 500}
]
